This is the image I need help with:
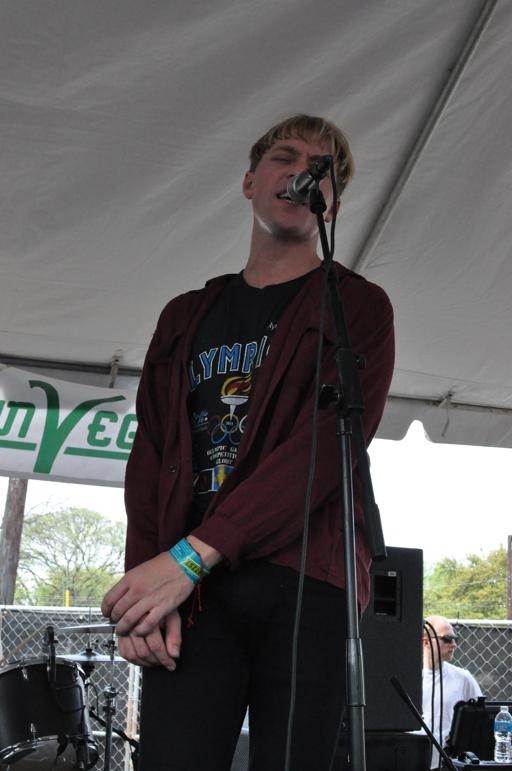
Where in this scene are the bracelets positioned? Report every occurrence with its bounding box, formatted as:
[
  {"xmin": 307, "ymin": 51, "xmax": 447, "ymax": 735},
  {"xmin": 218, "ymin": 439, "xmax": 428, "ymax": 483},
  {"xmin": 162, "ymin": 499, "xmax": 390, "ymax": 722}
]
[{"xmin": 168, "ymin": 538, "xmax": 211, "ymax": 586}]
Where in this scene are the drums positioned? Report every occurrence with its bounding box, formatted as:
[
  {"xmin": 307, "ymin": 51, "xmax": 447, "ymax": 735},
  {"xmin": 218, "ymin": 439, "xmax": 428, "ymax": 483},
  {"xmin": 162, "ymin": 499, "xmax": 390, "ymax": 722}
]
[{"xmin": 1, "ymin": 656, "xmax": 99, "ymax": 770}]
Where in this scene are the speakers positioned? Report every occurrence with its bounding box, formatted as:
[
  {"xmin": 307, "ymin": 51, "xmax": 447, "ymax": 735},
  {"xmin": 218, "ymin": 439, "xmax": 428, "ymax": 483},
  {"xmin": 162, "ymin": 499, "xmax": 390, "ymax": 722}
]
[
  {"xmin": 342, "ymin": 545, "xmax": 424, "ymax": 731},
  {"xmin": 340, "ymin": 731, "xmax": 433, "ymax": 770}
]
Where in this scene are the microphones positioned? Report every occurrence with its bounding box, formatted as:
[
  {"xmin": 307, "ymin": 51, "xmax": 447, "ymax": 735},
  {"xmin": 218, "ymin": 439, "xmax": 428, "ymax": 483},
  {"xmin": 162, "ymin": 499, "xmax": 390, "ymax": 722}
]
[
  {"xmin": 286, "ymin": 154, "xmax": 333, "ymax": 202},
  {"xmin": 390, "ymin": 674, "xmax": 458, "ymax": 771}
]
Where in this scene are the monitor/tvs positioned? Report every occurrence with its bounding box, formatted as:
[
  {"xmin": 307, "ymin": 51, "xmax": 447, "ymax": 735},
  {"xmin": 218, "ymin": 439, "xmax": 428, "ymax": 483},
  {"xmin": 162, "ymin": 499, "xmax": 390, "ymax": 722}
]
[{"xmin": 449, "ymin": 700, "xmax": 512, "ymax": 760}]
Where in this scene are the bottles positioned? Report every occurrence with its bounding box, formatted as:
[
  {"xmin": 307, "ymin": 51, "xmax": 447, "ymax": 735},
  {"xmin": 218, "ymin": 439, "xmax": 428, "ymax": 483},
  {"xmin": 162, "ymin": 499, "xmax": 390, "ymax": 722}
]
[{"xmin": 494, "ymin": 705, "xmax": 512, "ymax": 763}]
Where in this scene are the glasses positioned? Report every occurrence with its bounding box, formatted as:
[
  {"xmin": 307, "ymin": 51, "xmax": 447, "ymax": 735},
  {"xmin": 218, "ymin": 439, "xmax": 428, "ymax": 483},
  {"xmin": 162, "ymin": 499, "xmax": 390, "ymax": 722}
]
[{"xmin": 431, "ymin": 635, "xmax": 459, "ymax": 644}]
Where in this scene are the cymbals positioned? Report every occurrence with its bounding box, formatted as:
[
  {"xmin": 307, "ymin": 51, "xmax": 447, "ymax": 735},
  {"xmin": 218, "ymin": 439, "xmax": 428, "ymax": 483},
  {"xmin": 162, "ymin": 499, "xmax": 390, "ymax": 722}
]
[
  {"xmin": 55, "ymin": 624, "xmax": 117, "ymax": 633},
  {"xmin": 57, "ymin": 651, "xmax": 129, "ymax": 662}
]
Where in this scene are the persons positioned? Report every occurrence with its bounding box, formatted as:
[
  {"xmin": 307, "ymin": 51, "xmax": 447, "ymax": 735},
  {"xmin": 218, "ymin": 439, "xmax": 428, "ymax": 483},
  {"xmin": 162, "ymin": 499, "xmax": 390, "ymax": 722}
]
[
  {"xmin": 102, "ymin": 114, "xmax": 396, "ymax": 771},
  {"xmin": 422, "ymin": 615, "xmax": 483, "ymax": 768}
]
